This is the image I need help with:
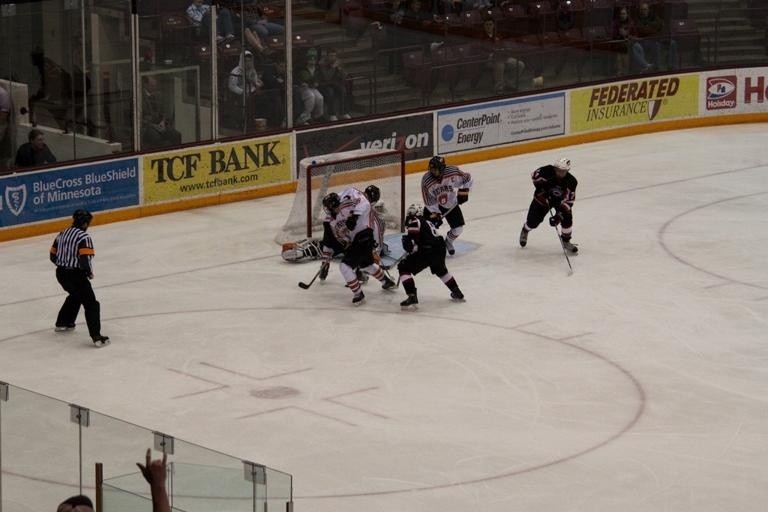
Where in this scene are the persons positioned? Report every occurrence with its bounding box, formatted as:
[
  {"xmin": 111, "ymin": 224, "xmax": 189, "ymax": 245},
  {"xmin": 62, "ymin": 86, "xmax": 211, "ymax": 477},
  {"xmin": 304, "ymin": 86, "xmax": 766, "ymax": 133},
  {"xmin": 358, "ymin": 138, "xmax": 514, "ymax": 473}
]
[
  {"xmin": 49, "ymin": 208, "xmax": 110, "ymax": 344},
  {"xmin": 27, "ymin": 45, "xmax": 91, "ymax": 100},
  {"xmin": 136, "ymin": 447, "xmax": 171, "ymax": 512},
  {"xmin": 149, "ymin": 113, "xmax": 181, "ymax": 146},
  {"xmin": 56, "ymin": 494, "xmax": 95, "ymax": 512},
  {"xmin": 187, "ymin": 1, "xmax": 701, "ymax": 135},
  {"xmin": 283, "ymin": 155, "xmax": 475, "ymax": 310},
  {"xmin": 13, "ymin": 129, "xmax": 57, "ymax": 170},
  {"xmin": 141, "ymin": 75, "xmax": 161, "ymax": 145},
  {"xmin": 519, "ymin": 155, "xmax": 578, "ymax": 255},
  {"xmin": 0, "ymin": 86, "xmax": 18, "ymax": 169}
]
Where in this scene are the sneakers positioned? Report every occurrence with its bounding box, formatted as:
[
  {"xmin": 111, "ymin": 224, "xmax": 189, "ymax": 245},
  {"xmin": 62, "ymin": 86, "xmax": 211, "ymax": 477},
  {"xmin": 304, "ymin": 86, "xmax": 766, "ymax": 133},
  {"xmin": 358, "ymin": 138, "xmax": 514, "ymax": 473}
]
[
  {"xmin": 343, "ymin": 276, "xmax": 464, "ymax": 307},
  {"xmin": 519, "ymin": 228, "xmax": 580, "ymax": 252},
  {"xmin": 52, "ymin": 316, "xmax": 112, "ymax": 343},
  {"xmin": 445, "ymin": 238, "xmax": 457, "ymax": 256}
]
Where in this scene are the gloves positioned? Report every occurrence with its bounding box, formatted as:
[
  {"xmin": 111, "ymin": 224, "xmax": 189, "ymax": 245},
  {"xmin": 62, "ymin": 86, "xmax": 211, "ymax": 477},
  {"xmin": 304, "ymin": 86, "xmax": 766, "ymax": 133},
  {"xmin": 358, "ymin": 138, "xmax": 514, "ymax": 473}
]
[
  {"xmin": 344, "ymin": 214, "xmax": 358, "ymax": 230},
  {"xmin": 456, "ymin": 188, "xmax": 470, "ymax": 204},
  {"xmin": 543, "ymin": 196, "xmax": 560, "ymax": 226},
  {"xmin": 429, "ymin": 212, "xmax": 443, "ymax": 229},
  {"xmin": 400, "ymin": 234, "xmax": 414, "ymax": 252}
]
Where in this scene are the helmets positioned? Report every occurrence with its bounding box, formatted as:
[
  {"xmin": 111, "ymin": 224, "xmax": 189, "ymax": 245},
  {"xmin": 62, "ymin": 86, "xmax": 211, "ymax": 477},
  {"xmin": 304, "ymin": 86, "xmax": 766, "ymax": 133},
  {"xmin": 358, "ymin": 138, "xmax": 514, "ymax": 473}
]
[
  {"xmin": 321, "ymin": 192, "xmax": 341, "ymax": 218},
  {"xmin": 365, "ymin": 185, "xmax": 385, "ymax": 214},
  {"xmin": 72, "ymin": 207, "xmax": 93, "ymax": 228},
  {"xmin": 554, "ymin": 157, "xmax": 571, "ymax": 177},
  {"xmin": 406, "ymin": 202, "xmax": 425, "ymax": 218},
  {"xmin": 429, "ymin": 155, "xmax": 446, "ymax": 182}
]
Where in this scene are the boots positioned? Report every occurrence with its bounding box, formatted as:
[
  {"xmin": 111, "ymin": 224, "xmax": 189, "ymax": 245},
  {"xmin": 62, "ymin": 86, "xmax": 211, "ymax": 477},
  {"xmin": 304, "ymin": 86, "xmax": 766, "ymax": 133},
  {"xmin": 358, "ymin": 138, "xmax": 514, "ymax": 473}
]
[{"xmin": 318, "ymin": 262, "xmax": 330, "ymax": 279}]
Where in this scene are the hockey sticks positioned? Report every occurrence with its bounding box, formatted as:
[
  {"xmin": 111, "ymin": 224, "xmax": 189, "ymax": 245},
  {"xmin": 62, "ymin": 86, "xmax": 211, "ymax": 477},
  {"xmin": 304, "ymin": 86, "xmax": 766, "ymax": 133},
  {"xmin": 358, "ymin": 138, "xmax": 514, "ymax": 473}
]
[
  {"xmin": 378, "ymin": 255, "xmax": 396, "ymax": 280},
  {"xmin": 392, "ymin": 275, "xmax": 401, "ymax": 289},
  {"xmin": 380, "ymin": 251, "xmax": 408, "ymax": 269},
  {"xmin": 298, "ymin": 251, "xmax": 335, "ymax": 289},
  {"xmin": 547, "ymin": 200, "xmax": 573, "ymax": 276}
]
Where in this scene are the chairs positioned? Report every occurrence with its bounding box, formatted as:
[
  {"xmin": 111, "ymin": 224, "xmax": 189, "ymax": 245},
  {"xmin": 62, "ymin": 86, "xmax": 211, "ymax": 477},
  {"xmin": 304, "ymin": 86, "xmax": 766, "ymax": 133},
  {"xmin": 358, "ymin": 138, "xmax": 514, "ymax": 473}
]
[{"xmin": 136, "ymin": 7, "xmax": 376, "ymax": 125}]
